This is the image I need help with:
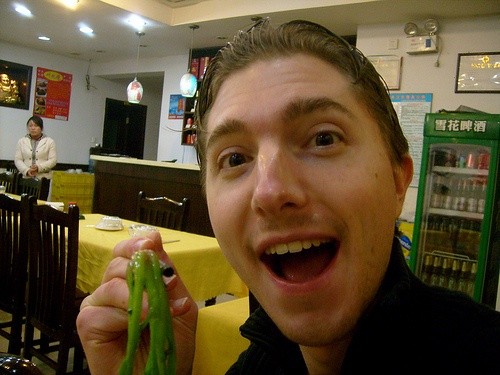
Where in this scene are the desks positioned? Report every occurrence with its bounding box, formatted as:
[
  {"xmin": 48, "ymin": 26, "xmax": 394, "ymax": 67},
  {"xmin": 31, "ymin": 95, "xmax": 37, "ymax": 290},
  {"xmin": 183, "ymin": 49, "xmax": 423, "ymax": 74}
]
[
  {"xmin": 45, "ymin": 170, "xmax": 95, "ymax": 215},
  {"xmin": 0, "ymin": 213, "xmax": 248, "ymax": 375},
  {"xmin": 192, "ymin": 293, "xmax": 249, "ymax": 375}
]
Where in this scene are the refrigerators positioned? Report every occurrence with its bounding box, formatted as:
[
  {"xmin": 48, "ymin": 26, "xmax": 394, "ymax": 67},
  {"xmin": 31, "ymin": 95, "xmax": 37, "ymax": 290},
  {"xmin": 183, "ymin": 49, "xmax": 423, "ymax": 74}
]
[{"xmin": 410, "ymin": 113, "xmax": 500, "ymax": 311}]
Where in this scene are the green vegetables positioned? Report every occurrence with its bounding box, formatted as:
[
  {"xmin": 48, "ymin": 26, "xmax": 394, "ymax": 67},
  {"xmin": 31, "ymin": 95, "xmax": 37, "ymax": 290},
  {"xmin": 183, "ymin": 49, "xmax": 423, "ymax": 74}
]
[{"xmin": 118, "ymin": 249, "xmax": 177, "ymax": 375}]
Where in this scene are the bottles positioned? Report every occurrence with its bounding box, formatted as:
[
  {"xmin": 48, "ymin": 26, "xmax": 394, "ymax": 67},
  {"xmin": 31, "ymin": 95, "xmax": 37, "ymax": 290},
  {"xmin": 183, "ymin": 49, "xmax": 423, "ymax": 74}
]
[
  {"xmin": 68, "ymin": 201, "xmax": 78, "ymax": 206},
  {"xmin": 421, "ymin": 176, "xmax": 487, "ymax": 297}
]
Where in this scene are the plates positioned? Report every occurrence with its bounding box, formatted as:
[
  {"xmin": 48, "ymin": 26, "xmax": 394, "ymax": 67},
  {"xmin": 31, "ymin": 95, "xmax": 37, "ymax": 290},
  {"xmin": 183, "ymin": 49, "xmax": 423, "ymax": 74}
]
[
  {"xmin": 129, "ymin": 224, "xmax": 159, "ymax": 238},
  {"xmin": 35, "ymin": 79, "xmax": 48, "ymax": 115},
  {"xmin": 97, "ymin": 223, "xmax": 123, "ymax": 230}
]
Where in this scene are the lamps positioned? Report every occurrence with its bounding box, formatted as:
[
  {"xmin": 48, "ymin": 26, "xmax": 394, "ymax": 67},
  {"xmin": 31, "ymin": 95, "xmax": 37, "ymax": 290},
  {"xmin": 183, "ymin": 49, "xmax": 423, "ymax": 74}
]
[
  {"xmin": 401, "ymin": 19, "xmax": 441, "ymax": 53},
  {"xmin": 180, "ymin": 26, "xmax": 199, "ymax": 98},
  {"xmin": 126, "ymin": 31, "xmax": 145, "ymax": 104}
]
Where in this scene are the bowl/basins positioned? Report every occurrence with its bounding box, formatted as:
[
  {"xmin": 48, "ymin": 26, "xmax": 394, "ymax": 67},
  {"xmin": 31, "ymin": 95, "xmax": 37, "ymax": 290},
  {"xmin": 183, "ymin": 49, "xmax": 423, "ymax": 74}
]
[{"xmin": 102, "ymin": 217, "xmax": 121, "ymax": 227}]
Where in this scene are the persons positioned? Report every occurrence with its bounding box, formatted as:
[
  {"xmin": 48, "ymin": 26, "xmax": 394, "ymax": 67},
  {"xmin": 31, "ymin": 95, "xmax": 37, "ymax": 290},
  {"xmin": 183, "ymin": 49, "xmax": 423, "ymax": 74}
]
[
  {"xmin": 76, "ymin": 17, "xmax": 500, "ymax": 375},
  {"xmin": 14, "ymin": 116, "xmax": 57, "ymax": 179}
]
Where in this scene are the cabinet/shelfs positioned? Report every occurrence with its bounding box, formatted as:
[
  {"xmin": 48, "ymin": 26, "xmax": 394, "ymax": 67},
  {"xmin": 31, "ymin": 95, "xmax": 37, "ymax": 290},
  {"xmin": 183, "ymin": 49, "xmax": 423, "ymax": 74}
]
[{"xmin": 181, "ymin": 35, "xmax": 357, "ymax": 145}]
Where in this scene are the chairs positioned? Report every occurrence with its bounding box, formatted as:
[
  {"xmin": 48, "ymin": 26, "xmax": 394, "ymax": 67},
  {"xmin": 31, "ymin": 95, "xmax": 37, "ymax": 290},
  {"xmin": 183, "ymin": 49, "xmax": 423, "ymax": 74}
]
[
  {"xmin": 133, "ymin": 192, "xmax": 188, "ymax": 231},
  {"xmin": 0, "ymin": 172, "xmax": 89, "ymax": 375}
]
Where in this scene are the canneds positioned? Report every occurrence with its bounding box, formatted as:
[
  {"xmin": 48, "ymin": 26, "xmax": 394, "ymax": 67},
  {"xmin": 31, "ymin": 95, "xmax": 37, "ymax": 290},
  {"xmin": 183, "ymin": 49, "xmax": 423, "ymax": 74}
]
[
  {"xmin": 443, "ymin": 149, "xmax": 489, "ymax": 170},
  {"xmin": 187, "ymin": 118, "xmax": 192, "ymax": 125},
  {"xmin": 186, "ymin": 133, "xmax": 196, "ymax": 144}
]
